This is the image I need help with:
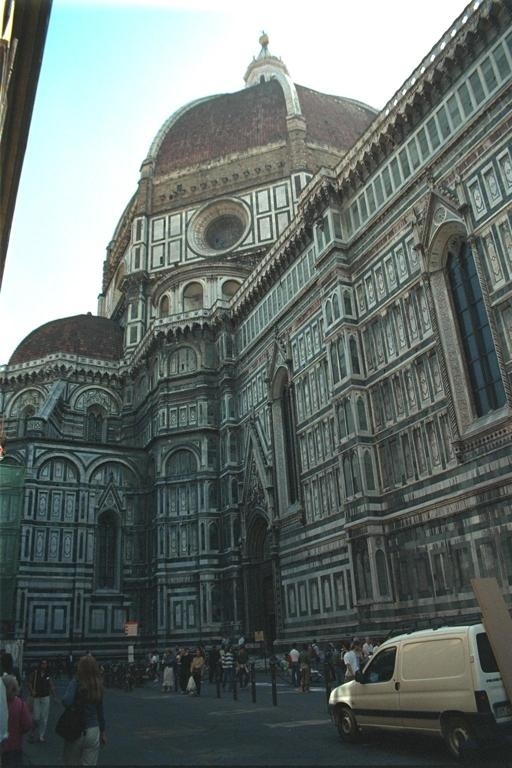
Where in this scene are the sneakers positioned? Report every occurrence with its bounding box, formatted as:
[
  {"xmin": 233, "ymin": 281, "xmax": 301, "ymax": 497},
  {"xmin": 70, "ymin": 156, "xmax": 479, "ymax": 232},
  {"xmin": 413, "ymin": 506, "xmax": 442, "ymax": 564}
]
[
  {"xmin": 26, "ymin": 736, "xmax": 34, "ymax": 743},
  {"xmin": 37, "ymin": 736, "xmax": 45, "ymax": 742}
]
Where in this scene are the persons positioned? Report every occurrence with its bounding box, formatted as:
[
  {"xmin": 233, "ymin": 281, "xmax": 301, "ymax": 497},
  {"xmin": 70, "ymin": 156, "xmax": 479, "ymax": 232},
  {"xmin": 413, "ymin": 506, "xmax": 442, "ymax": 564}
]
[
  {"xmin": 97, "ymin": 635, "xmax": 383, "ymax": 695},
  {"xmin": 0, "ymin": 650, "xmax": 108, "ymax": 768}
]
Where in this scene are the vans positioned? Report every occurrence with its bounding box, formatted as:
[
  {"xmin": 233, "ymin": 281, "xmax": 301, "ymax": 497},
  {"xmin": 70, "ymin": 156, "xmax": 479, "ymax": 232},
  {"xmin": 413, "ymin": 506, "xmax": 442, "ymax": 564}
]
[{"xmin": 326, "ymin": 621, "xmax": 511, "ymax": 758}]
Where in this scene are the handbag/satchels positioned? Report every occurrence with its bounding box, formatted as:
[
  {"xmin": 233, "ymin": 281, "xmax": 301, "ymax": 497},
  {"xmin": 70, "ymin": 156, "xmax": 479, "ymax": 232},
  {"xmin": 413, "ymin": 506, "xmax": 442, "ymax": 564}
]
[{"xmin": 54, "ymin": 708, "xmax": 84, "ymax": 742}]
